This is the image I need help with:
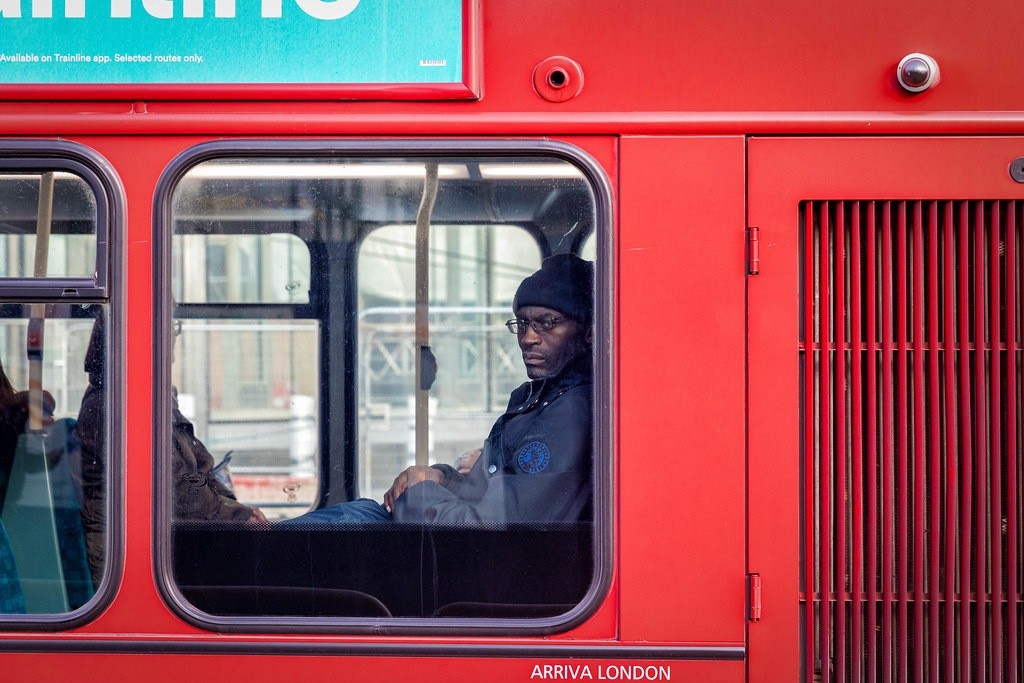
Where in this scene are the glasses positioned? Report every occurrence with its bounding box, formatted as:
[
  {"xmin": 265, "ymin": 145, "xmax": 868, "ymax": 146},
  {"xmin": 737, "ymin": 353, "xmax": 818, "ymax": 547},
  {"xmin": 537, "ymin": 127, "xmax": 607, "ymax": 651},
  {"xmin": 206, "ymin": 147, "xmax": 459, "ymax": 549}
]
[
  {"xmin": 505, "ymin": 315, "xmax": 570, "ymax": 334},
  {"xmin": 170, "ymin": 319, "xmax": 182, "ymax": 336}
]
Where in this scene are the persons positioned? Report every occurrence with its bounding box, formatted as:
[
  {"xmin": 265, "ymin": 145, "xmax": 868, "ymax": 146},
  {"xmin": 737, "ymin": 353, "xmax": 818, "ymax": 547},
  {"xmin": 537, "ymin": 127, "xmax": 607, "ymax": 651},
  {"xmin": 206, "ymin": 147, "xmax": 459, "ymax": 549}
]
[
  {"xmin": 0, "ymin": 359, "xmax": 56, "ymax": 517},
  {"xmin": 75, "ymin": 294, "xmax": 271, "ymax": 592},
  {"xmin": 381, "ymin": 253, "xmax": 594, "ymax": 524}
]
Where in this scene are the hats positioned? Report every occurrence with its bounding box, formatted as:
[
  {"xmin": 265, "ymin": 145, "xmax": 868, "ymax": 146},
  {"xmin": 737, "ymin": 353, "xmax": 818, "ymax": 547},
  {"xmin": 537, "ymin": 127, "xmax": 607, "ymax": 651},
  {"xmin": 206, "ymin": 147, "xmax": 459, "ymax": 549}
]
[
  {"xmin": 15, "ymin": 389, "xmax": 56, "ymax": 417},
  {"xmin": 513, "ymin": 254, "xmax": 594, "ymax": 330}
]
